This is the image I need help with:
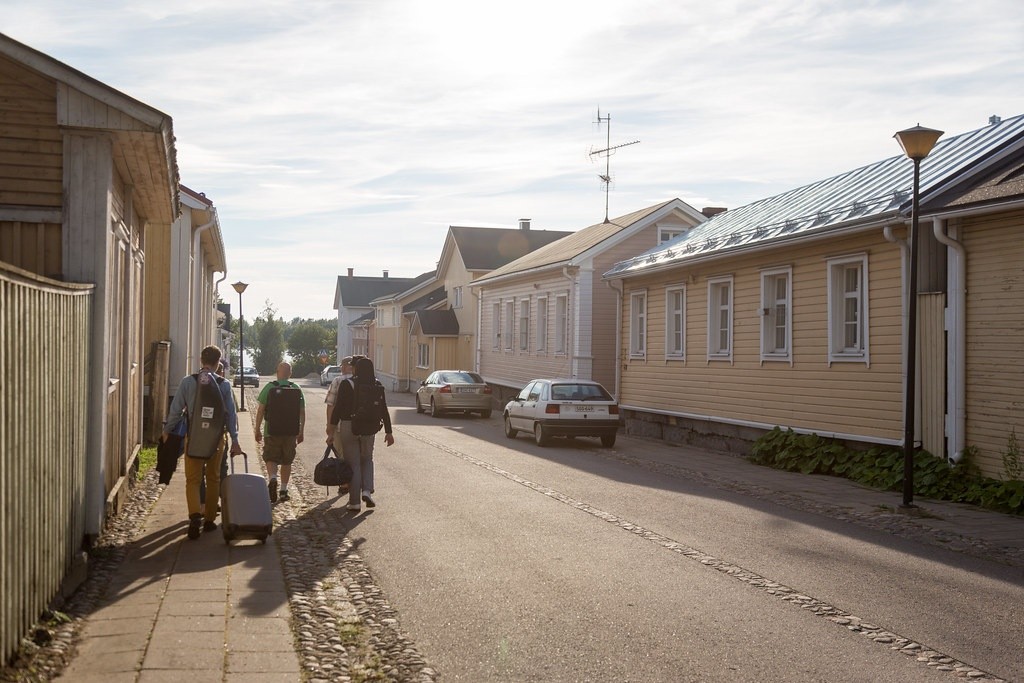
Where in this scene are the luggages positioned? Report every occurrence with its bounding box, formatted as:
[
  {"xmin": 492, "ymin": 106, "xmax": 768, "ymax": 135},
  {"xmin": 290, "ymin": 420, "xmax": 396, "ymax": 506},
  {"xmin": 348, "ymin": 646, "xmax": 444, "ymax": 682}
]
[{"xmin": 221, "ymin": 451, "xmax": 272, "ymax": 545}]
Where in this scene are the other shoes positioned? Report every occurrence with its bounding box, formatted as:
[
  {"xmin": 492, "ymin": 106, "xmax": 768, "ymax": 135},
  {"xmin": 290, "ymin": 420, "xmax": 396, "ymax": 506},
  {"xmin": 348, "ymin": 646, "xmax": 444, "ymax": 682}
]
[{"xmin": 337, "ymin": 487, "xmax": 347, "ymax": 494}]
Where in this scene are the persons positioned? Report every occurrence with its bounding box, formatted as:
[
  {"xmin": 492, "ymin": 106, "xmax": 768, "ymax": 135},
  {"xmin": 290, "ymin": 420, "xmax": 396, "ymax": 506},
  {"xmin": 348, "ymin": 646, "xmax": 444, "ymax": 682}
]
[
  {"xmin": 184, "ymin": 362, "xmax": 239, "ymax": 517},
  {"xmin": 324, "ymin": 354, "xmax": 369, "ymax": 495},
  {"xmin": 341, "ymin": 357, "xmax": 394, "ymax": 512},
  {"xmin": 254, "ymin": 362, "xmax": 305, "ymax": 504},
  {"xmin": 161, "ymin": 345, "xmax": 241, "ymax": 542}
]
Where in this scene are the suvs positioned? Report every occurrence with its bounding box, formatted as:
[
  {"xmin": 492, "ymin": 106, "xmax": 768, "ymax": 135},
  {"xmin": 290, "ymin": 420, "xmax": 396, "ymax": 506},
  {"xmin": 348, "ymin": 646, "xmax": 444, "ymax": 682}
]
[{"xmin": 319, "ymin": 364, "xmax": 342, "ymax": 386}]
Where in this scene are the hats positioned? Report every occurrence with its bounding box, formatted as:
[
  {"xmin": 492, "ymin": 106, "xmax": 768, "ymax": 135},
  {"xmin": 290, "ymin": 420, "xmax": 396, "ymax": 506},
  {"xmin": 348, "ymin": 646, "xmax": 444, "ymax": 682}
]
[
  {"xmin": 348, "ymin": 355, "xmax": 367, "ymax": 365},
  {"xmin": 340, "ymin": 356, "xmax": 353, "ymax": 366}
]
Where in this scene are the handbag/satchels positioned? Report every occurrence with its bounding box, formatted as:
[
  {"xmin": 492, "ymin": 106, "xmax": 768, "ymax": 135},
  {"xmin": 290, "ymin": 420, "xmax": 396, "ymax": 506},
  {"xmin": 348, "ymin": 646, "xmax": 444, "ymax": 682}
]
[{"xmin": 314, "ymin": 444, "xmax": 353, "ymax": 486}]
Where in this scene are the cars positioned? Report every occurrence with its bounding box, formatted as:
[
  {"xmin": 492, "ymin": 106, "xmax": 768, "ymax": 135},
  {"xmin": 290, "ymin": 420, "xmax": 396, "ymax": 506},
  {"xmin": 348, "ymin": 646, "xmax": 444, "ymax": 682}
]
[
  {"xmin": 234, "ymin": 366, "xmax": 261, "ymax": 387},
  {"xmin": 415, "ymin": 370, "xmax": 494, "ymax": 419},
  {"xmin": 502, "ymin": 379, "xmax": 624, "ymax": 449}
]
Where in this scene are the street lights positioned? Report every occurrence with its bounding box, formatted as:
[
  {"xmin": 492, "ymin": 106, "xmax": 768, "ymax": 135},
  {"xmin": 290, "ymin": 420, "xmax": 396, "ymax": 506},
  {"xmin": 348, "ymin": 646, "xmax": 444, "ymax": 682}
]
[
  {"xmin": 892, "ymin": 124, "xmax": 946, "ymax": 507},
  {"xmin": 230, "ymin": 280, "xmax": 250, "ymax": 412}
]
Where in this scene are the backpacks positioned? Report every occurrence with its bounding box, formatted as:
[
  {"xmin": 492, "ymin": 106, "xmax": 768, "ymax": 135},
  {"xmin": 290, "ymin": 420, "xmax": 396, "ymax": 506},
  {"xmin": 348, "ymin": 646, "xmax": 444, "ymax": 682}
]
[
  {"xmin": 189, "ymin": 374, "xmax": 225, "ymax": 459},
  {"xmin": 267, "ymin": 380, "xmax": 302, "ymax": 436}
]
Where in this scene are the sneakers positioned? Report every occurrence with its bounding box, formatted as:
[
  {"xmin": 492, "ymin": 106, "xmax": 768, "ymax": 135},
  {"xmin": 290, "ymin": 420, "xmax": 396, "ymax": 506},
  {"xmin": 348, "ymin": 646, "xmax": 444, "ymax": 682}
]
[
  {"xmin": 362, "ymin": 491, "xmax": 375, "ymax": 508},
  {"xmin": 280, "ymin": 490, "xmax": 290, "ymax": 500},
  {"xmin": 268, "ymin": 478, "xmax": 278, "ymax": 503},
  {"xmin": 188, "ymin": 517, "xmax": 199, "ymax": 539},
  {"xmin": 347, "ymin": 504, "xmax": 360, "ymax": 512},
  {"xmin": 204, "ymin": 522, "xmax": 217, "ymax": 531}
]
